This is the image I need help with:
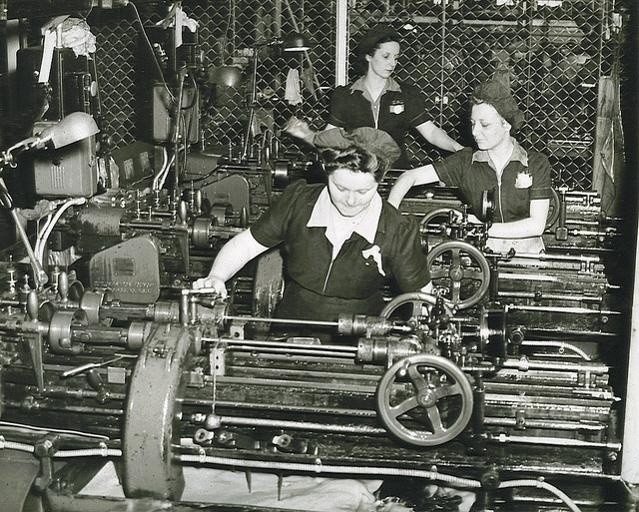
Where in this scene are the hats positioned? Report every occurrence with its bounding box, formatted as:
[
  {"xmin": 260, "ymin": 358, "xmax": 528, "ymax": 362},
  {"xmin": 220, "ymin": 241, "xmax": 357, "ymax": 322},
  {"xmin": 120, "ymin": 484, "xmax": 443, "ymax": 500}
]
[
  {"xmin": 312, "ymin": 127, "xmax": 401, "ymax": 166},
  {"xmin": 472, "ymin": 79, "xmax": 525, "ymax": 131},
  {"xmin": 359, "ymin": 26, "xmax": 399, "ymax": 56}
]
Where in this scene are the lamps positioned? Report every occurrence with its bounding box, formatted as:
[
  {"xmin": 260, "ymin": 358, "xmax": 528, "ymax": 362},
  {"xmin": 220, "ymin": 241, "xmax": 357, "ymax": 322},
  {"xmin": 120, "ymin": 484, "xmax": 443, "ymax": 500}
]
[
  {"xmin": 167, "ymin": 63, "xmax": 242, "ymax": 198},
  {"xmin": 239, "ymin": 32, "xmax": 313, "ymax": 162},
  {"xmin": 1, "ymin": 111, "xmax": 100, "ymax": 299}
]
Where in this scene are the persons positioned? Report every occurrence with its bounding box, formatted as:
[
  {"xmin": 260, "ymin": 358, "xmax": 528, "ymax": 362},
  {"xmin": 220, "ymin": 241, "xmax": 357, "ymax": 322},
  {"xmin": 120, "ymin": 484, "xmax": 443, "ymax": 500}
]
[
  {"xmin": 283, "ymin": 23, "xmax": 465, "ymax": 168},
  {"xmin": 388, "ymin": 79, "xmax": 553, "ymax": 253},
  {"xmin": 191, "ymin": 128, "xmax": 435, "ymax": 347}
]
